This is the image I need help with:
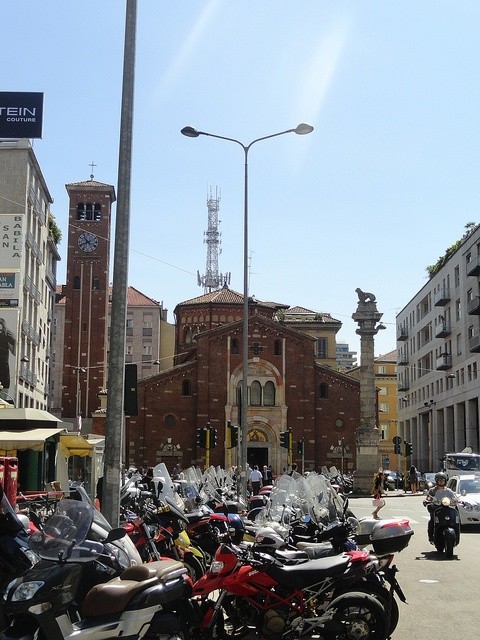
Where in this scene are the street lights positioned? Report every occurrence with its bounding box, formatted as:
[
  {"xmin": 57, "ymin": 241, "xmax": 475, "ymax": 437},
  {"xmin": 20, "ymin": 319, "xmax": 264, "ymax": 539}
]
[
  {"xmin": 181, "ymin": 124, "xmax": 313, "ymax": 498},
  {"xmin": 75, "ymin": 368, "xmax": 86, "ymax": 431}
]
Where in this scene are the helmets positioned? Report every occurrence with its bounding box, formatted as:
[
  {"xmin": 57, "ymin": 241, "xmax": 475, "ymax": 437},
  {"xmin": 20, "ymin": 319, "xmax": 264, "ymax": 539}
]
[{"xmin": 435, "ymin": 472, "xmax": 447, "ymax": 482}]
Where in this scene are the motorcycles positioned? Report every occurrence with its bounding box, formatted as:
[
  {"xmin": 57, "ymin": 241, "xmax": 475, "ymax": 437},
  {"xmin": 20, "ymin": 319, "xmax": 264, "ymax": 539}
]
[
  {"xmin": 268, "ymin": 518, "xmax": 379, "ymax": 551},
  {"xmin": 246, "ymin": 519, "xmax": 414, "ymax": 636},
  {"xmin": 0, "ymin": 499, "xmax": 193, "ymax": 640},
  {"xmin": 28, "ymin": 481, "xmax": 190, "ymax": 562},
  {"xmin": 121, "ymin": 462, "xmax": 358, "ymax": 523},
  {"xmin": 190, "ymin": 543, "xmax": 390, "ymax": 640},
  {"xmin": 423, "ymin": 489, "xmax": 466, "ymax": 558},
  {"xmin": 0, "ymin": 493, "xmax": 41, "ymax": 590}
]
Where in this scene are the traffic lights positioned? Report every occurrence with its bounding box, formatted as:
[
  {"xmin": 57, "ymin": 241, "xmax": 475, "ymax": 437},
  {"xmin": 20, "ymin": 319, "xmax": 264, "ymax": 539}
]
[
  {"xmin": 406, "ymin": 443, "xmax": 413, "ymax": 456},
  {"xmin": 196, "ymin": 427, "xmax": 206, "ymax": 448},
  {"xmin": 297, "ymin": 441, "xmax": 302, "ymax": 454},
  {"xmin": 280, "ymin": 431, "xmax": 289, "ymax": 448},
  {"xmin": 210, "ymin": 428, "xmax": 218, "ymax": 448},
  {"xmin": 231, "ymin": 426, "xmax": 238, "ymax": 447}
]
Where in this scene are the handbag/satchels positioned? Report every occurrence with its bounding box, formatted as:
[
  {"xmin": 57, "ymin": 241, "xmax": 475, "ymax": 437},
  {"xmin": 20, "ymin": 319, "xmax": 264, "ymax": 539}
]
[{"xmin": 373, "ymin": 494, "xmax": 383, "ymax": 506}]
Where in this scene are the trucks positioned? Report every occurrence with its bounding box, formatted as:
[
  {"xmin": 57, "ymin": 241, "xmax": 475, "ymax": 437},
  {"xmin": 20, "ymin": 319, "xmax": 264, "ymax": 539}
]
[{"xmin": 440, "ymin": 453, "xmax": 480, "ymax": 480}]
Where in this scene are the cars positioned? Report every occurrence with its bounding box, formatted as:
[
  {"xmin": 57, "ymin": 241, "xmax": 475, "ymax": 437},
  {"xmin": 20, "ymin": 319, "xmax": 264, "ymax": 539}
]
[
  {"xmin": 384, "ymin": 472, "xmax": 403, "ymax": 488},
  {"xmin": 445, "ymin": 474, "xmax": 480, "ymax": 529},
  {"xmin": 402, "ymin": 471, "xmax": 421, "ymax": 490},
  {"xmin": 419, "ymin": 473, "xmax": 437, "ymax": 491}
]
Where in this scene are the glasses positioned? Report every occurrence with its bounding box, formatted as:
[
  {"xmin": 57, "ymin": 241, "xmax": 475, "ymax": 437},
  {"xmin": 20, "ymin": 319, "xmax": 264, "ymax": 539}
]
[{"xmin": 438, "ymin": 478, "xmax": 445, "ymax": 481}]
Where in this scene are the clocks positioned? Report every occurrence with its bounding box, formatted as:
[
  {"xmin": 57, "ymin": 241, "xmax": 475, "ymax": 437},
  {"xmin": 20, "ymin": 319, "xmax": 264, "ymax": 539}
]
[{"xmin": 77, "ymin": 232, "xmax": 98, "ymax": 253}]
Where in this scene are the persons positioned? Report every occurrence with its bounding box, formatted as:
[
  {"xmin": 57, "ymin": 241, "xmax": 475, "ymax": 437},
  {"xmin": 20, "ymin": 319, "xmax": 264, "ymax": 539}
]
[
  {"xmin": 282, "ymin": 468, "xmax": 287, "ymax": 475},
  {"xmin": 409, "ymin": 465, "xmax": 417, "ymax": 493},
  {"xmin": 0, "ymin": 317, "xmax": 17, "ymax": 400},
  {"xmin": 268, "ymin": 466, "xmax": 274, "ymax": 474},
  {"xmin": 372, "ymin": 471, "xmax": 385, "ymax": 519},
  {"xmin": 230, "ymin": 465, "xmax": 241, "ymax": 490},
  {"xmin": 249, "ymin": 465, "xmax": 263, "ymax": 496},
  {"xmin": 173, "ymin": 464, "xmax": 181, "ymax": 480},
  {"xmin": 425, "ymin": 471, "xmax": 461, "ymax": 544},
  {"xmin": 247, "ymin": 463, "xmax": 253, "ymax": 496},
  {"xmin": 287, "ymin": 463, "xmax": 292, "ymax": 475},
  {"xmin": 96, "ymin": 477, "xmax": 103, "ymax": 511},
  {"xmin": 262, "ymin": 465, "xmax": 272, "ymax": 486},
  {"xmin": 140, "ymin": 467, "xmax": 154, "ymax": 485},
  {"xmin": 288, "ymin": 463, "xmax": 301, "ymax": 477}
]
